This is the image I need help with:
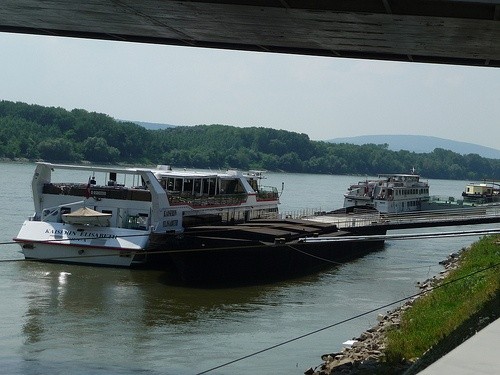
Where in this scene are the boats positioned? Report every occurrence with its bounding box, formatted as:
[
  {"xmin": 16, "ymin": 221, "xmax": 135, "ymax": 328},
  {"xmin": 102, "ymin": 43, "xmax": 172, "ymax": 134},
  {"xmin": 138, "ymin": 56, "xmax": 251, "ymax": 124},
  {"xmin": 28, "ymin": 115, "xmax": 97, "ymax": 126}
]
[
  {"xmin": 11, "ymin": 162, "xmax": 284, "ymax": 269},
  {"xmin": 342, "ymin": 169, "xmax": 431, "ymax": 214},
  {"xmin": 461, "ymin": 179, "xmax": 500, "ymax": 204}
]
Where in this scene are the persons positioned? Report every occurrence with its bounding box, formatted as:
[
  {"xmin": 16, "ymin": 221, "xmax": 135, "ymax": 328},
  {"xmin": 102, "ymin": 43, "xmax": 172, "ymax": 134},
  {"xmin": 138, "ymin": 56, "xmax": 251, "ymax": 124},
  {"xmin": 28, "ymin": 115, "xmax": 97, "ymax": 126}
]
[
  {"xmin": 168, "ymin": 180, "xmax": 174, "ymax": 191},
  {"xmin": 91, "ymin": 177, "xmax": 96, "ymax": 185}
]
